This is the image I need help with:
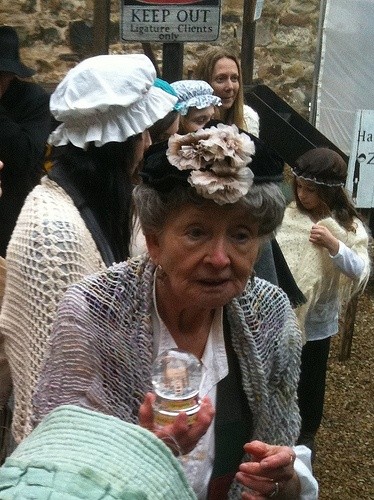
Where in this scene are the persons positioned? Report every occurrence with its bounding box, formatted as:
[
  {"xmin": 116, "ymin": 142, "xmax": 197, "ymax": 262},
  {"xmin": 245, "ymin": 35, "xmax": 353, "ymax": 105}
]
[{"xmin": 0, "ymin": 24, "xmax": 374, "ymax": 500}]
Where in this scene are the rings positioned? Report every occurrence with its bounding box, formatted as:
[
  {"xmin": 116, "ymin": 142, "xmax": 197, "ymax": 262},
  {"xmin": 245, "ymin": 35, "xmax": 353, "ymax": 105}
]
[
  {"xmin": 269, "ymin": 482, "xmax": 280, "ymax": 499},
  {"xmin": 317, "ymin": 236, "xmax": 321, "ymax": 240}
]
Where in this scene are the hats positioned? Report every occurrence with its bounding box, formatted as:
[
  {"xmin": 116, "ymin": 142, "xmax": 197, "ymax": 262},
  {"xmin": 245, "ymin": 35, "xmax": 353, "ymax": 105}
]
[
  {"xmin": 239, "ymin": 104, "xmax": 261, "ymax": 140},
  {"xmin": 0, "ymin": 26, "xmax": 37, "ymax": 79},
  {"xmin": 140, "ymin": 120, "xmax": 285, "ymax": 191},
  {"xmin": 152, "ymin": 78, "xmax": 185, "ymax": 113},
  {"xmin": 169, "ymin": 80, "xmax": 222, "ymax": 116},
  {"xmin": 46, "ymin": 53, "xmax": 179, "ymax": 152}
]
